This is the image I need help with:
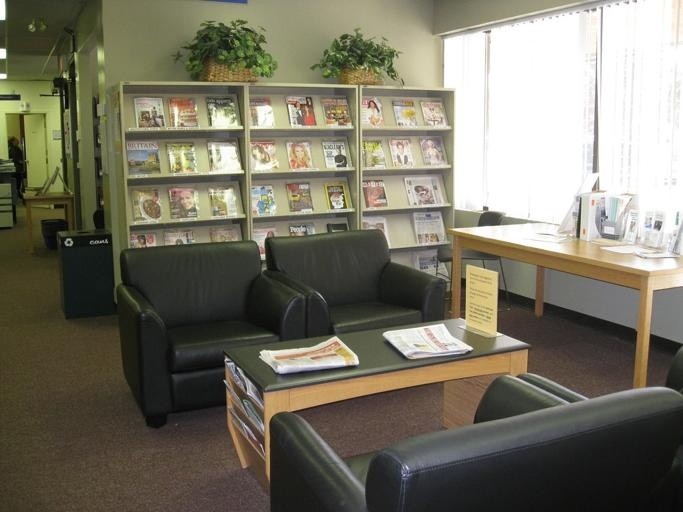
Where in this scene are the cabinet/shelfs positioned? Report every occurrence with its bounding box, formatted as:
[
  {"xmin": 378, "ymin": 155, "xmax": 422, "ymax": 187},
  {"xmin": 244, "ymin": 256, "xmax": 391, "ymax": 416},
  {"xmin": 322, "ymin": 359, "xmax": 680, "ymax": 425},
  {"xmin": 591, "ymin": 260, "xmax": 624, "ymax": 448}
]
[
  {"xmin": 0, "ymin": 159, "xmax": 18, "ymax": 224},
  {"xmin": 244, "ymin": 79, "xmax": 362, "ymax": 269},
  {"xmin": 106, "ymin": 76, "xmax": 249, "ymax": 302},
  {"xmin": 359, "ymin": 83, "xmax": 459, "ymax": 289}
]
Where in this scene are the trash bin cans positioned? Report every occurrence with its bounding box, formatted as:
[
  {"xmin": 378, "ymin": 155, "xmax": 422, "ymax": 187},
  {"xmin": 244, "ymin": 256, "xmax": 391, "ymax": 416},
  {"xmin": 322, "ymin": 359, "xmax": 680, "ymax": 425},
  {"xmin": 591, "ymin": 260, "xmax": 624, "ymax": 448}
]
[{"xmin": 41, "ymin": 220, "xmax": 68, "ymax": 248}]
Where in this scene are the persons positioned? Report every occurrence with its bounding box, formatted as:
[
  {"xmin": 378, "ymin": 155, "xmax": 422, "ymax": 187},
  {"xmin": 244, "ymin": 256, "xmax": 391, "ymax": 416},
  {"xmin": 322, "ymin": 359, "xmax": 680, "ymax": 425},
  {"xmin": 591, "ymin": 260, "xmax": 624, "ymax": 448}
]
[{"xmin": 7, "ymin": 136, "xmax": 25, "ymax": 199}]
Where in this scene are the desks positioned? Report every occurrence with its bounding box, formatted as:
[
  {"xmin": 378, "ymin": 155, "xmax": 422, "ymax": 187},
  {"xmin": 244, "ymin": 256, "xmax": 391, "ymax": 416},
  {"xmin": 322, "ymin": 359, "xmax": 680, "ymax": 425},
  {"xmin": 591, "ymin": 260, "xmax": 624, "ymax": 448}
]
[
  {"xmin": 445, "ymin": 220, "xmax": 681, "ymax": 391},
  {"xmin": 24, "ymin": 191, "xmax": 76, "ymax": 253}
]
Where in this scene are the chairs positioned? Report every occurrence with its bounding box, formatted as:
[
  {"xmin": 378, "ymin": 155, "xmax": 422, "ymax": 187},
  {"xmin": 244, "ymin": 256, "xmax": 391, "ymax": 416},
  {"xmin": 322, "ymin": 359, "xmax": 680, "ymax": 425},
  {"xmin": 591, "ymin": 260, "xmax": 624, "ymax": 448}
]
[
  {"xmin": 114, "ymin": 239, "xmax": 306, "ymax": 429},
  {"xmin": 517, "ymin": 345, "xmax": 682, "ymax": 432},
  {"xmin": 267, "ymin": 371, "xmax": 681, "ymax": 511},
  {"xmin": 431, "ymin": 211, "xmax": 513, "ymax": 311},
  {"xmin": 262, "ymin": 228, "xmax": 446, "ymax": 336}
]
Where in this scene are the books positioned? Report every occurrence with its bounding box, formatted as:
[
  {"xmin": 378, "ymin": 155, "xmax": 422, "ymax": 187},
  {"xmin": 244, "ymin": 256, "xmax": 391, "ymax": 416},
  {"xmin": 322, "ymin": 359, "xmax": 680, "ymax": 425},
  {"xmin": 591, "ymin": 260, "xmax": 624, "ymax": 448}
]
[
  {"xmin": 126, "ymin": 95, "xmax": 245, "ymax": 247},
  {"xmin": 571, "ymin": 192, "xmax": 638, "ymax": 244},
  {"xmin": 249, "ymin": 97, "xmax": 354, "ymax": 260},
  {"xmin": 222, "ymin": 358, "xmax": 265, "ymax": 459},
  {"xmin": 361, "ymin": 97, "xmax": 448, "ymax": 280}
]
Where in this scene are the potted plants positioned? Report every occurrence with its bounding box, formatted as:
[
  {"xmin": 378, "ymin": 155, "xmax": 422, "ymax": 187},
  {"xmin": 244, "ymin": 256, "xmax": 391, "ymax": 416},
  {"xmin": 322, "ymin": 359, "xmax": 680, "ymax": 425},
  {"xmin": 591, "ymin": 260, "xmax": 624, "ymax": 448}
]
[
  {"xmin": 307, "ymin": 29, "xmax": 400, "ymax": 87},
  {"xmin": 173, "ymin": 19, "xmax": 278, "ymax": 84}
]
